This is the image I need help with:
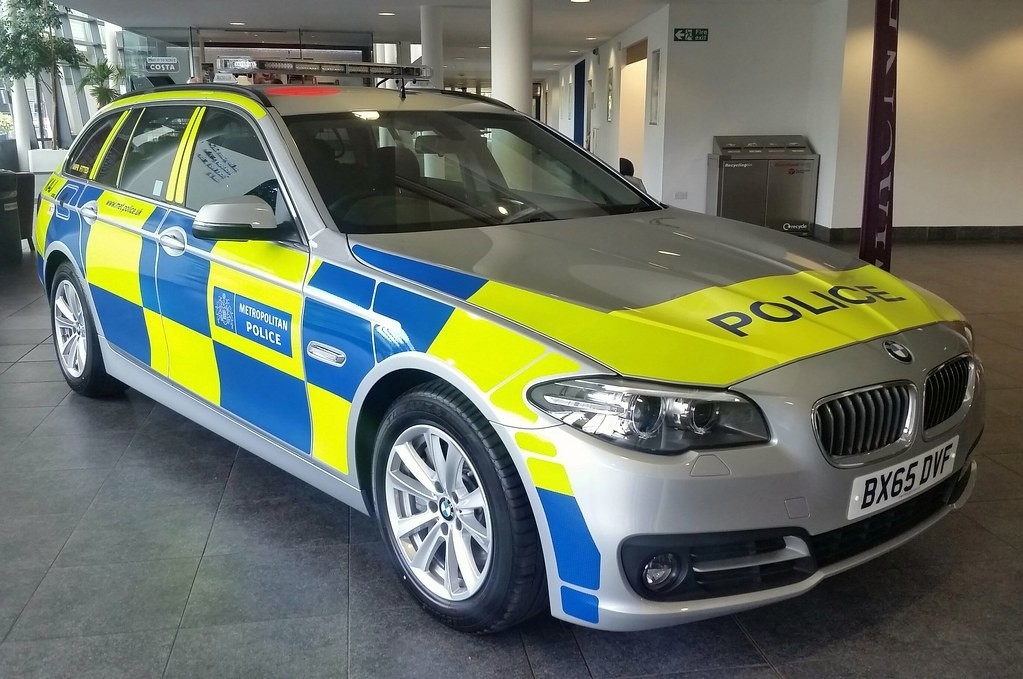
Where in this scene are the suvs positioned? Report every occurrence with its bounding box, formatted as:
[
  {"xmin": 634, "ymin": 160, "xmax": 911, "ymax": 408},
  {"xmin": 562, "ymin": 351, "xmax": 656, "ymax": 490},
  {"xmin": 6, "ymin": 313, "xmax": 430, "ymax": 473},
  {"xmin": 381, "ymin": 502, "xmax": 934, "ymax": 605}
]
[{"xmin": 31, "ymin": 55, "xmax": 987, "ymax": 637}]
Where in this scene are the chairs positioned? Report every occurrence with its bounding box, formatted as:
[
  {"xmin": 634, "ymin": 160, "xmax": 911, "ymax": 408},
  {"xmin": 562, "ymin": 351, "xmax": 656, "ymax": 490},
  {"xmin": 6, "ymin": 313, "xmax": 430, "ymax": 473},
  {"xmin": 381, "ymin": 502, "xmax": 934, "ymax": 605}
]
[
  {"xmin": 14, "ymin": 172, "xmax": 35, "ymax": 251},
  {"xmin": 371, "ymin": 147, "xmax": 421, "ymax": 195}
]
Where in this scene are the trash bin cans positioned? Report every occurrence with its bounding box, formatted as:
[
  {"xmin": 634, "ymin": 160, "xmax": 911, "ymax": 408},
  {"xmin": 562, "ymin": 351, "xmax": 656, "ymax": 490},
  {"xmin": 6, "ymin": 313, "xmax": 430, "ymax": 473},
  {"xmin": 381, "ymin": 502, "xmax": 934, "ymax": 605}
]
[{"xmin": 0, "ymin": 169, "xmax": 23, "ymax": 266}]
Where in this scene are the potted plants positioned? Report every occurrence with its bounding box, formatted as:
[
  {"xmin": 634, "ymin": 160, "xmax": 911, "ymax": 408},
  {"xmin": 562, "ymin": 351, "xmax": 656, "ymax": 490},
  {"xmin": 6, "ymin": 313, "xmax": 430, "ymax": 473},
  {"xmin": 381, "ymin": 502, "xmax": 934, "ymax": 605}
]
[{"xmin": 0, "ymin": 1, "xmax": 88, "ymax": 198}]
